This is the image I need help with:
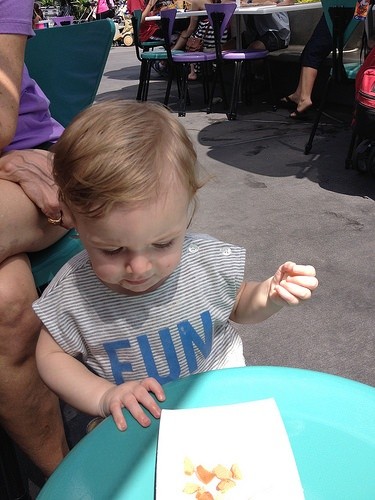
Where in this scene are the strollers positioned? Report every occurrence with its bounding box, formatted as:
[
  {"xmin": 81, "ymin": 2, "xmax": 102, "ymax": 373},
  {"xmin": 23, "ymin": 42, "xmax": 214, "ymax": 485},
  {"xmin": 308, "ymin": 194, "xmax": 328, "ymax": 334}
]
[{"xmin": 348, "ymin": 0, "xmax": 375, "ymax": 172}]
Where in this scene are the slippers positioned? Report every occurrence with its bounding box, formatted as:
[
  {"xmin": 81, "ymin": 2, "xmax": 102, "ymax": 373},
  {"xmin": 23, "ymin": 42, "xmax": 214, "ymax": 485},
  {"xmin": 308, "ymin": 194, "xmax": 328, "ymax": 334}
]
[
  {"xmin": 279, "ymin": 94, "xmax": 298, "ymax": 107},
  {"xmin": 288, "ymin": 109, "xmax": 318, "ymax": 120}
]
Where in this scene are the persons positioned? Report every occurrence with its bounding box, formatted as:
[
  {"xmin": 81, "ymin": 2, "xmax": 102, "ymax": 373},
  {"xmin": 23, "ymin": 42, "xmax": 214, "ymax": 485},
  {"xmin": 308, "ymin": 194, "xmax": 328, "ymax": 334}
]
[
  {"xmin": 0, "ymin": 0, "xmax": 68, "ymax": 478},
  {"xmin": 31, "ymin": 99, "xmax": 319, "ymax": 434},
  {"xmin": 32, "ymin": 0, "xmax": 356, "ymax": 118}
]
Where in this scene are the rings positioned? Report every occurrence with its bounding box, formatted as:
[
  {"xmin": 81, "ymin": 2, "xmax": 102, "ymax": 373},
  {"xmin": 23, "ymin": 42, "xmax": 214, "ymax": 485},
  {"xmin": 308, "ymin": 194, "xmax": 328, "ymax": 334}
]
[{"xmin": 48, "ymin": 217, "xmax": 61, "ymax": 224}]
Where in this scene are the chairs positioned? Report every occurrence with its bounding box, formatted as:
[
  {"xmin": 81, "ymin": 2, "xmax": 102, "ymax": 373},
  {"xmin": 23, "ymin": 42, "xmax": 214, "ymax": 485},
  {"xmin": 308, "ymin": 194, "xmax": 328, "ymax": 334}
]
[
  {"xmin": 132, "ymin": 0, "xmax": 375, "ymax": 175},
  {"xmin": 24, "ymin": 19, "xmax": 116, "ymax": 289}
]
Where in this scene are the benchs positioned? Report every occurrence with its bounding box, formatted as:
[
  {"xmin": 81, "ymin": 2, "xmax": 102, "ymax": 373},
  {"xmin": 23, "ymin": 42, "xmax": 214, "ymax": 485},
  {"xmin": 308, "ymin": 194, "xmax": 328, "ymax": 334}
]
[{"xmin": 268, "ymin": 8, "xmax": 364, "ymax": 64}]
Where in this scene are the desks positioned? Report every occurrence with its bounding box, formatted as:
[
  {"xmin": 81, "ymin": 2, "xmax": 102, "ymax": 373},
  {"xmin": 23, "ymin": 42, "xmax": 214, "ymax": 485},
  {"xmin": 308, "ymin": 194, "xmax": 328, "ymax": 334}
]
[
  {"xmin": 30, "ymin": 366, "xmax": 374, "ymax": 500},
  {"xmin": 145, "ymin": 2, "xmax": 322, "ymax": 106}
]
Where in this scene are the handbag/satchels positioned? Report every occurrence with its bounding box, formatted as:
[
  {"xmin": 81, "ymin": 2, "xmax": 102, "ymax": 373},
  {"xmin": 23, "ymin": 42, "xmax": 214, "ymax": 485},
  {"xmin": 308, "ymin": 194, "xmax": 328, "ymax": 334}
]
[
  {"xmin": 355, "ymin": 45, "xmax": 375, "ymax": 110},
  {"xmin": 186, "ymin": 37, "xmax": 203, "ymax": 52}
]
